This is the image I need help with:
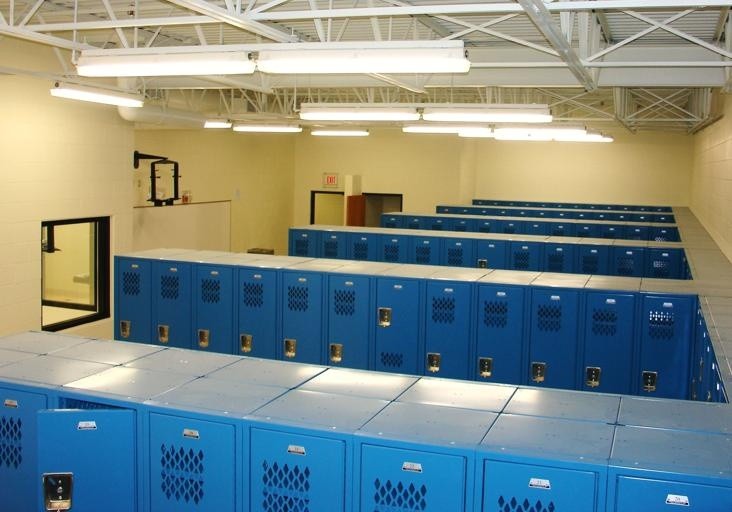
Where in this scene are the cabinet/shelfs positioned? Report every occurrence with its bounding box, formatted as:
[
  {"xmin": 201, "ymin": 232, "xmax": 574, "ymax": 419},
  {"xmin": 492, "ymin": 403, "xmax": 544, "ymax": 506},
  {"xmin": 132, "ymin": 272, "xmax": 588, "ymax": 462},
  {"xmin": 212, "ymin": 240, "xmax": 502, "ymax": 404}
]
[
  {"xmin": 288, "ymin": 199, "xmax": 687, "ymax": 280},
  {"xmin": 114, "ymin": 249, "xmax": 708, "ymax": 400},
  {"xmin": 2, "ymin": 329, "xmax": 731, "ymax": 507}
]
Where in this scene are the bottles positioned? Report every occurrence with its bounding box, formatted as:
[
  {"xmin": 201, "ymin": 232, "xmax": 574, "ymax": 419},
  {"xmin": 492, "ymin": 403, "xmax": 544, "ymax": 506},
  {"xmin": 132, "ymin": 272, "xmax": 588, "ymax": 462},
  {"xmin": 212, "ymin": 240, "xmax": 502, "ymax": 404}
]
[{"xmin": 181, "ymin": 190, "xmax": 192, "ymax": 204}]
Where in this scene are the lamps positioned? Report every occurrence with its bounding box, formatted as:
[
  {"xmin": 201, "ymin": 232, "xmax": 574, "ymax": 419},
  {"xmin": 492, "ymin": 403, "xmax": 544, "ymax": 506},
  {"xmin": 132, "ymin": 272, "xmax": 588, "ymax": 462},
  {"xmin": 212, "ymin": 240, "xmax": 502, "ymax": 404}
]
[{"xmin": 50, "ymin": 39, "xmax": 614, "ymax": 143}]
